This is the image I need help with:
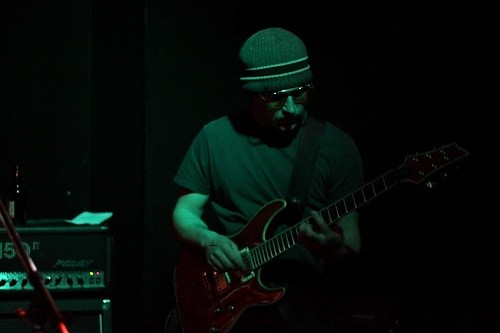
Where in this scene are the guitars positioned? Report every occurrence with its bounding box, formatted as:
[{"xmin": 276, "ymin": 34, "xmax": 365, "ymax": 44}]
[{"xmin": 174, "ymin": 142, "xmax": 470, "ymax": 333}]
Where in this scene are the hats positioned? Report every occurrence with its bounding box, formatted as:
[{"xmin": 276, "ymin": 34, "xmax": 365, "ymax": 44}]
[{"xmin": 240, "ymin": 28, "xmax": 312, "ymax": 91}]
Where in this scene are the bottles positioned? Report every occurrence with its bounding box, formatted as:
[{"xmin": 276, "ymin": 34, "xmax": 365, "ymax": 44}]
[{"xmin": 8, "ymin": 164, "xmax": 27, "ymax": 227}]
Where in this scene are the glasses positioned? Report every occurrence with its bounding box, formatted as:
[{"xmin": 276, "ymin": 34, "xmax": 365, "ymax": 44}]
[{"xmin": 258, "ymin": 83, "xmax": 312, "ymax": 100}]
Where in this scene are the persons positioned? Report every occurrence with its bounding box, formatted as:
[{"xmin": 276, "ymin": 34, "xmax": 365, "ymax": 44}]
[{"xmin": 171, "ymin": 27, "xmax": 362, "ymax": 333}]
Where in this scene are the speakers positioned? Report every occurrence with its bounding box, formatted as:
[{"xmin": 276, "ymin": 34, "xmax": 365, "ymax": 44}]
[{"xmin": 0, "ymin": 300, "xmax": 112, "ymax": 333}]
